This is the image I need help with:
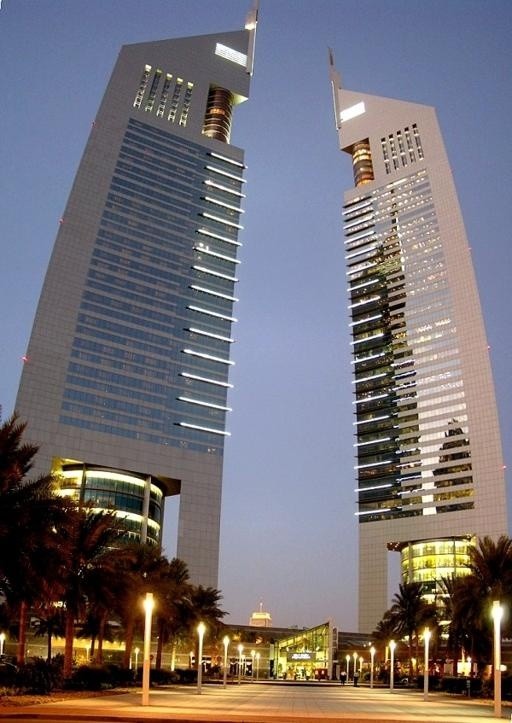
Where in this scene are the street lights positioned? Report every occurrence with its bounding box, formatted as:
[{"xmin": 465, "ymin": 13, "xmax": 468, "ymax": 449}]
[
  {"xmin": 86, "ymin": 644, "xmax": 89, "ymax": 661},
  {"xmin": 135, "ymin": 592, "xmax": 261, "ymax": 706},
  {"xmin": 345, "ymin": 600, "xmax": 504, "ymax": 717},
  {"xmin": 0, "ymin": 633, "xmax": 6, "ymax": 655}
]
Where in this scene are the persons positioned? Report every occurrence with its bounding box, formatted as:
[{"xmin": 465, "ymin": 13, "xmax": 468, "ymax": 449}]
[
  {"xmin": 353, "ymin": 670, "xmax": 359, "ymax": 687},
  {"xmin": 340, "ymin": 669, "xmax": 346, "ymax": 686}
]
